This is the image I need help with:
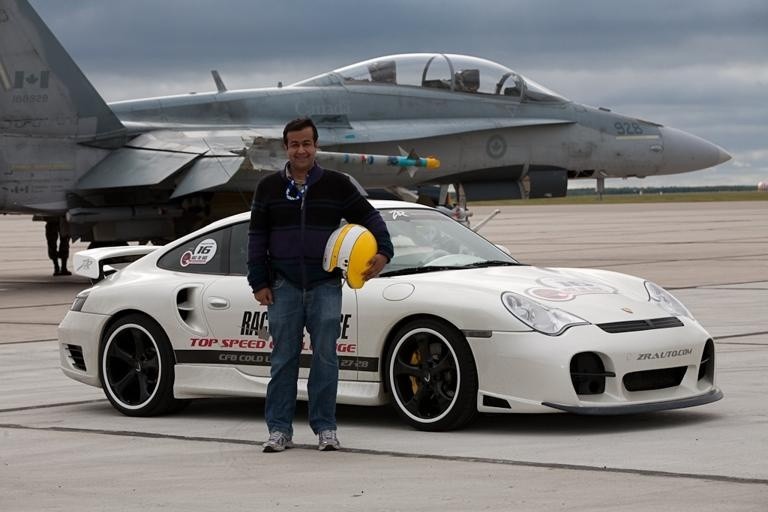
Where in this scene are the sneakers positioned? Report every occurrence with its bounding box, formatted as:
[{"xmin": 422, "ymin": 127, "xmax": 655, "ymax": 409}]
[
  {"xmin": 318, "ymin": 430, "xmax": 340, "ymax": 451},
  {"xmin": 262, "ymin": 431, "xmax": 294, "ymax": 452}
]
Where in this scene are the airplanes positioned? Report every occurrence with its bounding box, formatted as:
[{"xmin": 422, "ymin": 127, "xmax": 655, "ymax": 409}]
[{"xmin": 0, "ymin": 0, "xmax": 733, "ymax": 254}]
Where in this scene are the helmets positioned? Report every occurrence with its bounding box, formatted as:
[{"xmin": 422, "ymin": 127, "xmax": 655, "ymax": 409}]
[{"xmin": 322, "ymin": 223, "xmax": 377, "ymax": 289}]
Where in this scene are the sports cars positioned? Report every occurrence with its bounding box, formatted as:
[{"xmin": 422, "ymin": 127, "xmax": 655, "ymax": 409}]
[{"xmin": 57, "ymin": 200, "xmax": 726, "ymax": 431}]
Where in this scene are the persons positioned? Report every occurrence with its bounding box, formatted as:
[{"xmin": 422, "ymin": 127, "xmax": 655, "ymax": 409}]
[
  {"xmin": 455, "ymin": 69, "xmax": 469, "ymax": 91},
  {"xmin": 247, "ymin": 118, "xmax": 394, "ymax": 452},
  {"xmin": 45, "ymin": 217, "xmax": 71, "ymax": 276}
]
